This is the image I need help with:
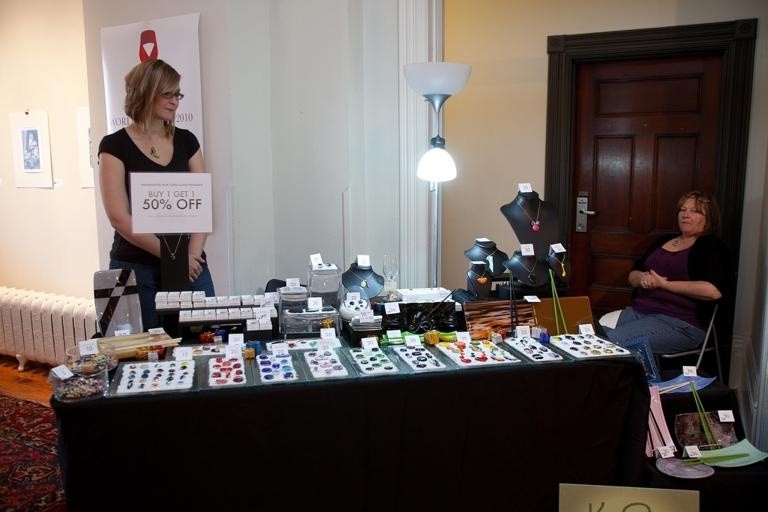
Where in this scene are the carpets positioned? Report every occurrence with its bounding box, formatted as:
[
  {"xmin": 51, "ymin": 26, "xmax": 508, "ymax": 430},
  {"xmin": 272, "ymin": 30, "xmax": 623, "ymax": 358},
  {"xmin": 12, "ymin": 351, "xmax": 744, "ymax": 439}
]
[{"xmin": 0, "ymin": 394, "xmax": 68, "ymax": 512}]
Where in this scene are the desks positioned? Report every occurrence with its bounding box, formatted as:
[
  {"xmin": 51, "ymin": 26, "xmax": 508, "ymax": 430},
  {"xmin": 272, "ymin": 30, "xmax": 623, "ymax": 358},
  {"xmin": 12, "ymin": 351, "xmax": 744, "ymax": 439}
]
[{"xmin": 51, "ymin": 336, "xmax": 637, "ymax": 512}]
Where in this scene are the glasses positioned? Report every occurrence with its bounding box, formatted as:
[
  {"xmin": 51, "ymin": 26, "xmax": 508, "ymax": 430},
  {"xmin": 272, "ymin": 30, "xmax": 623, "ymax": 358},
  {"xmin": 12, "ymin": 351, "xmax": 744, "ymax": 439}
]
[{"xmin": 160, "ymin": 92, "xmax": 184, "ymax": 100}]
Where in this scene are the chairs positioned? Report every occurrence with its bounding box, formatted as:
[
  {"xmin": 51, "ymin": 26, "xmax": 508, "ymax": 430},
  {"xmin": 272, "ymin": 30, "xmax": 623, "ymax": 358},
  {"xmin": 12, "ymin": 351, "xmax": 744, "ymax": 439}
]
[{"xmin": 660, "ymin": 299, "xmax": 722, "ymax": 386}]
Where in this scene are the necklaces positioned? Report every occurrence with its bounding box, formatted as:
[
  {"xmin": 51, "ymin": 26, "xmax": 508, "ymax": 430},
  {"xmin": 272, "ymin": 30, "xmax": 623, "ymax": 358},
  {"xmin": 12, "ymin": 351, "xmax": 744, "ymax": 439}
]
[
  {"xmin": 148, "ymin": 137, "xmax": 166, "ymax": 157},
  {"xmin": 161, "ymin": 233, "xmax": 180, "ymax": 261},
  {"xmin": 511, "ymin": 255, "xmax": 540, "ymax": 286},
  {"xmin": 468, "ymin": 269, "xmax": 489, "ymax": 284},
  {"xmin": 348, "ymin": 269, "xmax": 376, "ymax": 290},
  {"xmin": 671, "ymin": 236, "xmax": 682, "ymax": 247},
  {"xmin": 549, "ymin": 253, "xmax": 567, "ymax": 278},
  {"xmin": 516, "ymin": 198, "xmax": 543, "ymax": 233},
  {"xmin": 476, "ymin": 246, "xmax": 498, "ymax": 275}
]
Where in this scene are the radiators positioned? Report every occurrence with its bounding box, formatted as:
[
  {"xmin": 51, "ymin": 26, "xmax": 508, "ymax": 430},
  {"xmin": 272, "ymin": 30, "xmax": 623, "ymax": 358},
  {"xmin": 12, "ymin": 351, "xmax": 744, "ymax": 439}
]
[{"xmin": 0, "ymin": 287, "xmax": 98, "ymax": 373}]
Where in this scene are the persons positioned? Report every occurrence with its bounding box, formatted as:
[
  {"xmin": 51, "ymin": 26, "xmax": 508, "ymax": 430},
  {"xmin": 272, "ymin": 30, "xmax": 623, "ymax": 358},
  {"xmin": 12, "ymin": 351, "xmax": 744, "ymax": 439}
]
[
  {"xmin": 96, "ymin": 60, "xmax": 216, "ymax": 332},
  {"xmin": 592, "ymin": 189, "xmax": 728, "ymax": 354}
]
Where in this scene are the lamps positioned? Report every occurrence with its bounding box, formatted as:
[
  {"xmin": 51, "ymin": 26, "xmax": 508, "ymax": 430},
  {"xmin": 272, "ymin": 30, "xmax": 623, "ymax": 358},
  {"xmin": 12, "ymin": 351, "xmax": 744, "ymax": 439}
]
[{"xmin": 403, "ymin": 63, "xmax": 472, "ymax": 182}]
